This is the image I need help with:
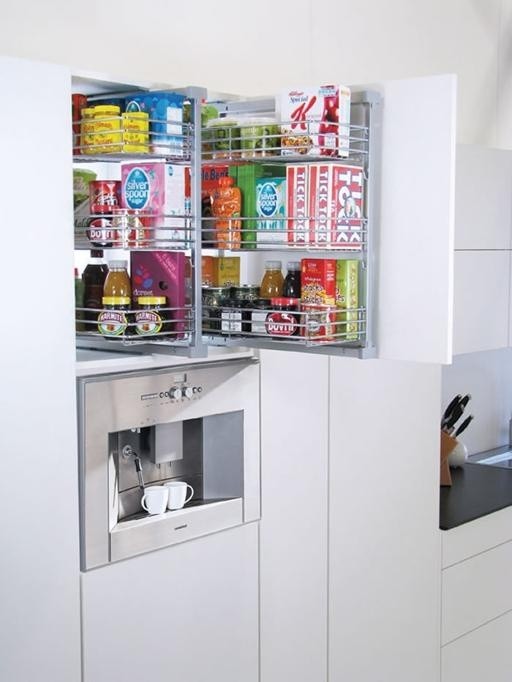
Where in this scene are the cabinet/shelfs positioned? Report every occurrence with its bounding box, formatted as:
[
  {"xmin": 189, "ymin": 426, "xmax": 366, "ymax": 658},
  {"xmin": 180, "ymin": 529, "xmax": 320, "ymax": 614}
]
[{"xmin": 0, "ymin": 64, "xmax": 512, "ymax": 682}]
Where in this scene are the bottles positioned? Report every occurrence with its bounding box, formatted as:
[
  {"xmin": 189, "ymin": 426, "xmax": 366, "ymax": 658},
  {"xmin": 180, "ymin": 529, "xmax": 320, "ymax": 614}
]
[
  {"xmin": 283, "ymin": 260, "xmax": 301, "ymax": 298},
  {"xmin": 84, "ymin": 246, "xmax": 111, "ymax": 329},
  {"xmin": 261, "ymin": 261, "xmax": 284, "ymax": 298},
  {"xmin": 75, "ymin": 268, "xmax": 85, "ymax": 331},
  {"xmin": 212, "ymin": 176, "xmax": 242, "ymax": 250},
  {"xmin": 103, "ymin": 259, "xmax": 132, "ymax": 298}
]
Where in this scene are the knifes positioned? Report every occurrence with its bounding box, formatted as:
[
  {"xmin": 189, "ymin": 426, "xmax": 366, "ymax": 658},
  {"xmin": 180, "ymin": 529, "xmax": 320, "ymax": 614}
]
[{"xmin": 441, "ymin": 394, "xmax": 474, "ymax": 437}]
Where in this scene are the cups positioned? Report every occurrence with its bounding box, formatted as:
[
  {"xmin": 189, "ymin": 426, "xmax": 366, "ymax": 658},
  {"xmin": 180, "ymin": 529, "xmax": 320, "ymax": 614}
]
[
  {"xmin": 140, "ymin": 486, "xmax": 168, "ymax": 515},
  {"xmin": 164, "ymin": 480, "xmax": 194, "ymax": 510}
]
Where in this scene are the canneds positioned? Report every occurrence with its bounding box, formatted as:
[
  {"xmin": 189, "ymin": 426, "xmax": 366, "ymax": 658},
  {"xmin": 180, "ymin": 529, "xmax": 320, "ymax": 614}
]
[
  {"xmin": 201, "ymin": 284, "xmax": 231, "ymax": 335},
  {"xmin": 131, "ymin": 296, "xmax": 170, "ymax": 340},
  {"xmin": 229, "ymin": 284, "xmax": 260, "ymax": 336},
  {"xmin": 264, "ymin": 296, "xmax": 300, "ymax": 339},
  {"xmin": 86, "ymin": 180, "xmax": 151, "ymax": 247},
  {"xmin": 97, "ymin": 296, "xmax": 135, "ymax": 340},
  {"xmin": 80, "ymin": 105, "xmax": 122, "ymax": 155}
]
